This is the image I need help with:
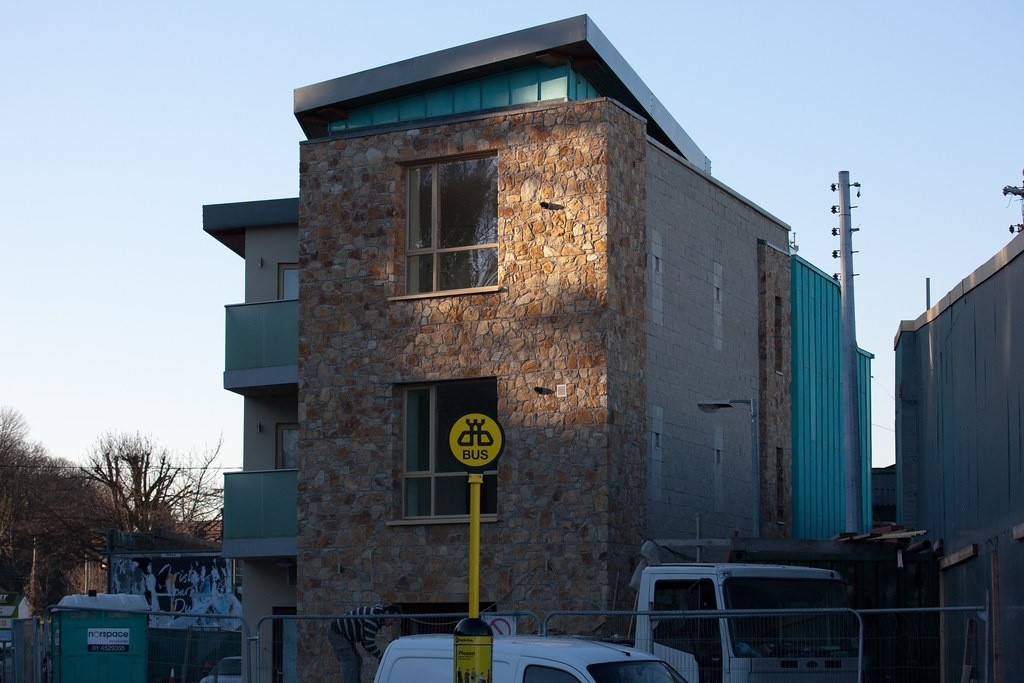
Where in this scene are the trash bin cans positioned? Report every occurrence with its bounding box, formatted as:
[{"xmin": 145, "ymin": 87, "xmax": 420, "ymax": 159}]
[{"xmin": 51, "ymin": 590, "xmax": 149, "ymax": 683}]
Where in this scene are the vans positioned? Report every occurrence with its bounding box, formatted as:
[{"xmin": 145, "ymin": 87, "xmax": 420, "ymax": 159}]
[{"xmin": 373, "ymin": 633, "xmax": 691, "ymax": 683}]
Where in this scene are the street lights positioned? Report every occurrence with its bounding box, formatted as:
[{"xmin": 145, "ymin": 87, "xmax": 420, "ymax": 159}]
[{"xmin": 699, "ymin": 396, "xmax": 766, "ymax": 536}]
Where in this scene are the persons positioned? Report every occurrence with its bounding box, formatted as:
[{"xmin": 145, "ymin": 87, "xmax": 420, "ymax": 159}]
[{"xmin": 327, "ymin": 602, "xmax": 402, "ymax": 683}]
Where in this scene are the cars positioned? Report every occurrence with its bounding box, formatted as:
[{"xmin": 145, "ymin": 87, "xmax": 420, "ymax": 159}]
[{"xmin": 200, "ymin": 656, "xmax": 242, "ymax": 683}]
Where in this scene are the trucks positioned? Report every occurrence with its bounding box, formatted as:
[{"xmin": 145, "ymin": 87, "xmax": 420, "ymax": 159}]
[{"xmin": 593, "ymin": 562, "xmax": 866, "ymax": 683}]
